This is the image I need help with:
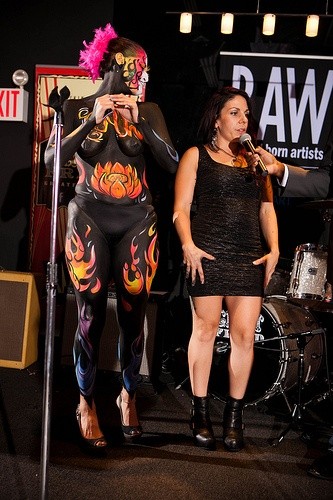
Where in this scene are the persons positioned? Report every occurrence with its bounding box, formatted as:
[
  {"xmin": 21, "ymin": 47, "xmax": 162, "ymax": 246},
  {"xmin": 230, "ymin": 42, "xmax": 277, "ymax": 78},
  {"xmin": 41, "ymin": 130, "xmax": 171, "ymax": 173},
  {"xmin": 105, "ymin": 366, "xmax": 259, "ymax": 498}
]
[
  {"xmin": 42, "ymin": 35, "xmax": 178, "ymax": 449},
  {"xmin": 247, "ymin": 145, "xmax": 332, "ymax": 200},
  {"xmin": 172, "ymin": 85, "xmax": 280, "ymax": 451}
]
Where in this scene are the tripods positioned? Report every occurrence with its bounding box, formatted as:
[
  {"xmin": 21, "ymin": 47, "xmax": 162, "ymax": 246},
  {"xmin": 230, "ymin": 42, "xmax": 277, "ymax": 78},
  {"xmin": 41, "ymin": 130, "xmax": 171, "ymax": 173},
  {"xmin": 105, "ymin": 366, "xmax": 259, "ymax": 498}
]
[{"xmin": 226, "ymin": 325, "xmax": 332, "ymax": 448}]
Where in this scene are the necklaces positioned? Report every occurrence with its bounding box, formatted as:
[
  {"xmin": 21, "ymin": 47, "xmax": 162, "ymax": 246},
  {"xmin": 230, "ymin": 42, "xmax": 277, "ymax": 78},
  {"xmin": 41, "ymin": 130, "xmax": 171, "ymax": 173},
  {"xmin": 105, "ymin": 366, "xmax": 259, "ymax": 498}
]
[{"xmin": 212, "ymin": 142, "xmax": 243, "ymax": 168}]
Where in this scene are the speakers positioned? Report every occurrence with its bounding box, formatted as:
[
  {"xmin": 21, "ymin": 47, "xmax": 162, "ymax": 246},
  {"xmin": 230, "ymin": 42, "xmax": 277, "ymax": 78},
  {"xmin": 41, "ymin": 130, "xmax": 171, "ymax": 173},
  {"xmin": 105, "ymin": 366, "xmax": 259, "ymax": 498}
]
[
  {"xmin": 0, "ymin": 270, "xmax": 42, "ymax": 369},
  {"xmin": 57, "ymin": 295, "xmax": 158, "ymax": 377}
]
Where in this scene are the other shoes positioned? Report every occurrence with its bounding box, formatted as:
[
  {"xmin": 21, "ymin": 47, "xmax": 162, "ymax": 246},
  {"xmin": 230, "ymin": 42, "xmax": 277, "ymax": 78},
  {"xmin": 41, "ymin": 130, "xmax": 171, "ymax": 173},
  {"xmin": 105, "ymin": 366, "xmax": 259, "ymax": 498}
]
[{"xmin": 307, "ymin": 467, "xmax": 333, "ymax": 478}]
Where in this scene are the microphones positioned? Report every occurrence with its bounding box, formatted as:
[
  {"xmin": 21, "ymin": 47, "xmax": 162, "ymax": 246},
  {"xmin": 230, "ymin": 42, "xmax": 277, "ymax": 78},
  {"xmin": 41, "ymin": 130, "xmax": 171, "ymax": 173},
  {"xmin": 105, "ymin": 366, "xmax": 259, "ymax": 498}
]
[{"xmin": 238, "ymin": 132, "xmax": 269, "ymax": 177}]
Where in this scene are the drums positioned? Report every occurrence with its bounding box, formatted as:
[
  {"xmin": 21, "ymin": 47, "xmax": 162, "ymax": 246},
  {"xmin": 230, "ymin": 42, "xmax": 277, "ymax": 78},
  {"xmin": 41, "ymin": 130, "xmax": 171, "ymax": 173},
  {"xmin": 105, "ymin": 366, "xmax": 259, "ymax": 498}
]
[
  {"xmin": 207, "ymin": 294, "xmax": 327, "ymax": 410},
  {"xmin": 285, "ymin": 243, "xmax": 330, "ymax": 309},
  {"xmin": 263, "ymin": 267, "xmax": 292, "ymax": 297}
]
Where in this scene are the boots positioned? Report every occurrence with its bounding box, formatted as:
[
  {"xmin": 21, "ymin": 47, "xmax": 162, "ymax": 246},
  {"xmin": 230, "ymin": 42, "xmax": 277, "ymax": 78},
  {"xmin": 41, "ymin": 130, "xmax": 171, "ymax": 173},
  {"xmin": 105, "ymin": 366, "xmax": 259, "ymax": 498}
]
[
  {"xmin": 190, "ymin": 395, "xmax": 217, "ymax": 449},
  {"xmin": 222, "ymin": 395, "xmax": 248, "ymax": 450}
]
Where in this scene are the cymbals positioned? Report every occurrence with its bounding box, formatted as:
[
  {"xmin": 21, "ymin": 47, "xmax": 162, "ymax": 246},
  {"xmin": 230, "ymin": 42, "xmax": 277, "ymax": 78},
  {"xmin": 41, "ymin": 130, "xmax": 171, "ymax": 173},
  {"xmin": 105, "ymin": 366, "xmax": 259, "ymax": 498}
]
[{"xmin": 305, "ymin": 199, "xmax": 333, "ymax": 209}]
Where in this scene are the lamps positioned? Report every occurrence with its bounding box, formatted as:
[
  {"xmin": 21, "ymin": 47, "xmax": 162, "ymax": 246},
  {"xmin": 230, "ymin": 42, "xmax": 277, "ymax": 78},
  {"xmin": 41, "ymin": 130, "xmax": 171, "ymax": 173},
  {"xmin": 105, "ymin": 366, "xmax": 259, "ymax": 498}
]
[
  {"xmin": 305, "ymin": 15, "xmax": 319, "ymax": 37},
  {"xmin": 262, "ymin": 13, "xmax": 275, "ymax": 36},
  {"xmin": 220, "ymin": 13, "xmax": 234, "ymax": 34},
  {"xmin": 0, "ymin": 70, "xmax": 30, "ymax": 123},
  {"xmin": 179, "ymin": 12, "xmax": 192, "ymax": 33}
]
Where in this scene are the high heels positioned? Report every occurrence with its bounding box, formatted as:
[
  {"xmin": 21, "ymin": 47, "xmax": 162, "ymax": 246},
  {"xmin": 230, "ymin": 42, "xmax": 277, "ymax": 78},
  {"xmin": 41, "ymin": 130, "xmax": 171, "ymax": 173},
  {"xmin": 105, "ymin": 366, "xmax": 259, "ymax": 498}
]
[
  {"xmin": 117, "ymin": 396, "xmax": 140, "ymax": 436},
  {"xmin": 74, "ymin": 404, "xmax": 108, "ymax": 448}
]
